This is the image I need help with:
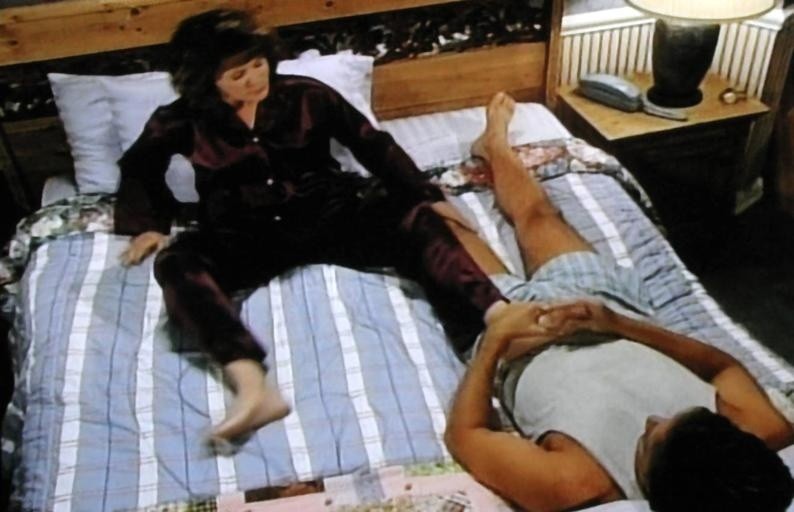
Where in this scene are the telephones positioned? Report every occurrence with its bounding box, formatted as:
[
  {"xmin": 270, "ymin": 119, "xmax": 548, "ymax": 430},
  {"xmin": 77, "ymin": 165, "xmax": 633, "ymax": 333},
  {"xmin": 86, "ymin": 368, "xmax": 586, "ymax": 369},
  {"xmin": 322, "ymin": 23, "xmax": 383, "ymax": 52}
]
[{"xmin": 574, "ymin": 73, "xmax": 644, "ymax": 111}]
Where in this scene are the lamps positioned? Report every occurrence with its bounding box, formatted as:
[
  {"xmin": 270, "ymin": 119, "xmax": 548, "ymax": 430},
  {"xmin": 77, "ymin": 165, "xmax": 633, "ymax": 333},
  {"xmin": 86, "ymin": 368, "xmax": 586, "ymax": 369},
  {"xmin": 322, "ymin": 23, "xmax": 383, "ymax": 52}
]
[{"xmin": 624, "ymin": 1, "xmax": 776, "ymax": 110}]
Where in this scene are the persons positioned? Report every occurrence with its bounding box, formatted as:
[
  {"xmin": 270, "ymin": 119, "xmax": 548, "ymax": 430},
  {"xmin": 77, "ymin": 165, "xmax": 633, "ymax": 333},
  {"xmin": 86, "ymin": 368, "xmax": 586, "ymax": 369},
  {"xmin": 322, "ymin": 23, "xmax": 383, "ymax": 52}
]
[
  {"xmin": 443, "ymin": 89, "xmax": 793, "ymax": 511},
  {"xmin": 112, "ymin": 7, "xmax": 592, "ymax": 446}
]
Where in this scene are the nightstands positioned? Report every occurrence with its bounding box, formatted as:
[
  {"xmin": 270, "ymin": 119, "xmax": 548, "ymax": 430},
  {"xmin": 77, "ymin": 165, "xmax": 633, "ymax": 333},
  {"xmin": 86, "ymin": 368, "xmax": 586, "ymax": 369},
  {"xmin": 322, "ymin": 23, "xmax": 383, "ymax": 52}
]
[{"xmin": 554, "ymin": 68, "xmax": 771, "ymax": 267}]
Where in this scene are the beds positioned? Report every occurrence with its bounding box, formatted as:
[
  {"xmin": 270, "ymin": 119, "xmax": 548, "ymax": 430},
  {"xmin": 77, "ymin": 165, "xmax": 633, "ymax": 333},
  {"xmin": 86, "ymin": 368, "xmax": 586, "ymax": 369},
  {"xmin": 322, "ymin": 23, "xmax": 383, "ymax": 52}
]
[{"xmin": 0, "ymin": 1, "xmax": 792, "ymax": 511}]
